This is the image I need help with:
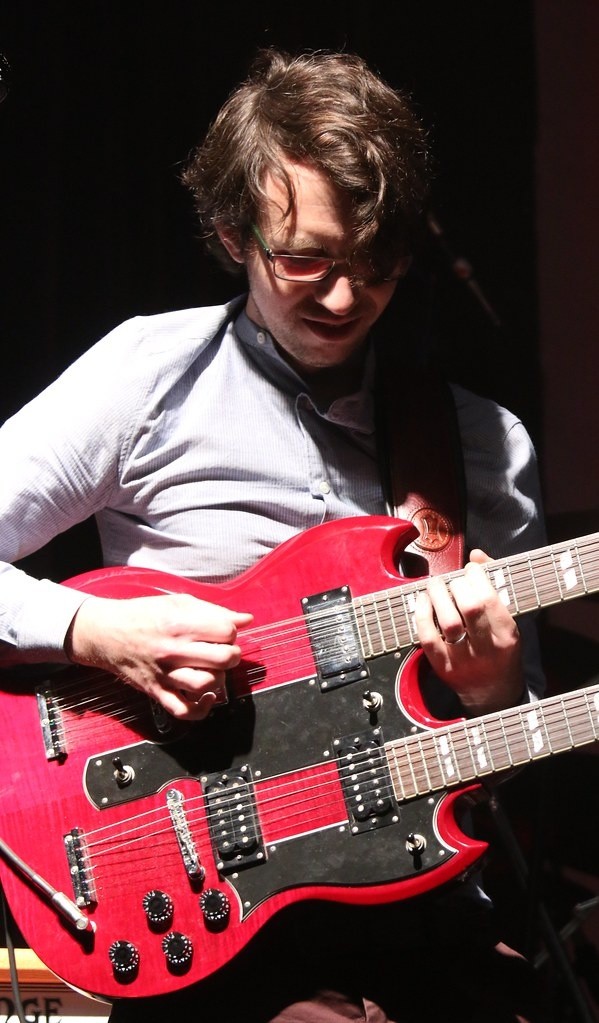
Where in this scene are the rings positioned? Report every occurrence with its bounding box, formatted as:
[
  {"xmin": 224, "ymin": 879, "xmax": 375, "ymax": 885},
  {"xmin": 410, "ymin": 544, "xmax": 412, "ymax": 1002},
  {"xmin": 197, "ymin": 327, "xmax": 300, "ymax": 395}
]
[{"xmin": 445, "ymin": 632, "xmax": 467, "ymax": 647}]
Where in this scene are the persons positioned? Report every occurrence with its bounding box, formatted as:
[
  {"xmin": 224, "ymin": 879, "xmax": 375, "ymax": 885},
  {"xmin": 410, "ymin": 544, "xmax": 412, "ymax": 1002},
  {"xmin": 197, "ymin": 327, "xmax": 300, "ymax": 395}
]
[{"xmin": 0, "ymin": 48, "xmax": 547, "ymax": 1023}]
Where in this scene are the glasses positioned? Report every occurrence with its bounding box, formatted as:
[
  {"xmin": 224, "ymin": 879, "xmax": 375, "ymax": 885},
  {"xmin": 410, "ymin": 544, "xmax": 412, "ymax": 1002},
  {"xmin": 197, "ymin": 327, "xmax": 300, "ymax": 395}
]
[{"xmin": 247, "ymin": 217, "xmax": 412, "ymax": 282}]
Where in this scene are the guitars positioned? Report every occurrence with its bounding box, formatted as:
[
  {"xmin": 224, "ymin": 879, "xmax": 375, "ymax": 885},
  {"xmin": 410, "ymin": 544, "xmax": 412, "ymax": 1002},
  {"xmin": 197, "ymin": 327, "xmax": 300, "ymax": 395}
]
[{"xmin": 0, "ymin": 514, "xmax": 599, "ymax": 1011}]
